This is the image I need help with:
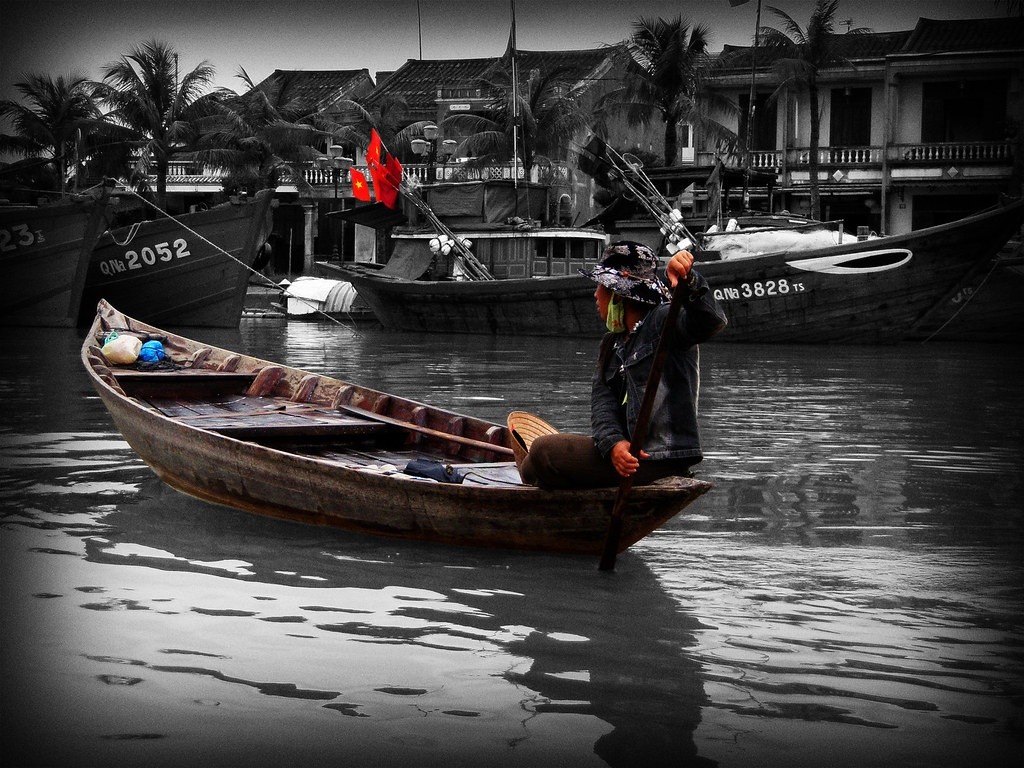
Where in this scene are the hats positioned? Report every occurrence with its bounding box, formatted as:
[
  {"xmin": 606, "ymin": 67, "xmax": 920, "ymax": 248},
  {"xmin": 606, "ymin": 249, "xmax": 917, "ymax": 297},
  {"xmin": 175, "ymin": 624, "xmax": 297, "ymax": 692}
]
[
  {"xmin": 506, "ymin": 410, "xmax": 562, "ymax": 475},
  {"xmin": 576, "ymin": 241, "xmax": 672, "ymax": 305}
]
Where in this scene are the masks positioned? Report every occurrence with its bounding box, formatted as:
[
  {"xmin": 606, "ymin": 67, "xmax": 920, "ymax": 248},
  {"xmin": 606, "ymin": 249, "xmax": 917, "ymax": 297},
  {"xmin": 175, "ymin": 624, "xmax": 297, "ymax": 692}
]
[{"xmin": 606, "ymin": 293, "xmax": 623, "ymax": 333}]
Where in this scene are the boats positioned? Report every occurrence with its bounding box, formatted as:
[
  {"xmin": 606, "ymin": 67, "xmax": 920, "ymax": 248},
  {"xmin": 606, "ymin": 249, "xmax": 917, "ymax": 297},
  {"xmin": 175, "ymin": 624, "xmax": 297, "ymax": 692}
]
[
  {"xmin": 241, "ymin": 275, "xmax": 377, "ymax": 323},
  {"xmin": 82, "ymin": 187, "xmax": 277, "ymax": 331},
  {"xmin": 0, "ymin": 177, "xmax": 118, "ymax": 329},
  {"xmin": 81, "ymin": 296, "xmax": 714, "ymax": 557},
  {"xmin": 315, "ymin": 195, "xmax": 1024, "ymax": 349}
]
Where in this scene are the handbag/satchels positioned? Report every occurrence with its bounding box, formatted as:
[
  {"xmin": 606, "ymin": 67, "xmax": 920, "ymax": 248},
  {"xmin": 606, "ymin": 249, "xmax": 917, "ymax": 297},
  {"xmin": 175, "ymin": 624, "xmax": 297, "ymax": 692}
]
[{"xmin": 404, "ymin": 458, "xmax": 465, "ymax": 484}]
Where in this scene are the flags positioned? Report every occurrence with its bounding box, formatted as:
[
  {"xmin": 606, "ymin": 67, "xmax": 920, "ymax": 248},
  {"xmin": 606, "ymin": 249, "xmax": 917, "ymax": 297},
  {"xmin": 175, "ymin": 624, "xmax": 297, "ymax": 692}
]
[{"xmin": 349, "ymin": 126, "xmax": 401, "ymax": 208}]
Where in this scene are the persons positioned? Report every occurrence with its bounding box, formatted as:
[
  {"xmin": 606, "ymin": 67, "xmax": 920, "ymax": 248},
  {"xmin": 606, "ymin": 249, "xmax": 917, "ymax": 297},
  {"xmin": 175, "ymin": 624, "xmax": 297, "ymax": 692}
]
[{"xmin": 520, "ymin": 241, "xmax": 728, "ymax": 492}]
